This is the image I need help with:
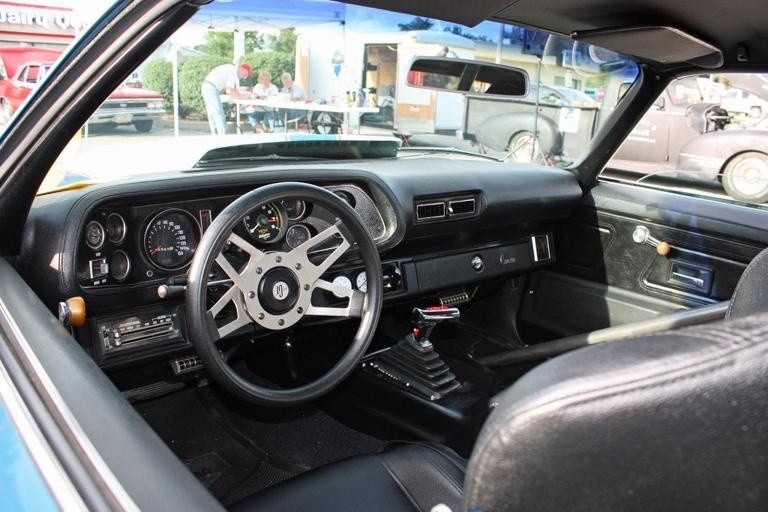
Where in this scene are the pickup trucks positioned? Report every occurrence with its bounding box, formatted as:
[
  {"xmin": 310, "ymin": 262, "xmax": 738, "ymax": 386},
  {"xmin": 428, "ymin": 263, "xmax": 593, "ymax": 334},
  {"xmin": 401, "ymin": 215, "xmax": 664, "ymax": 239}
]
[{"xmin": 463, "ymin": 75, "xmax": 767, "ymax": 206}]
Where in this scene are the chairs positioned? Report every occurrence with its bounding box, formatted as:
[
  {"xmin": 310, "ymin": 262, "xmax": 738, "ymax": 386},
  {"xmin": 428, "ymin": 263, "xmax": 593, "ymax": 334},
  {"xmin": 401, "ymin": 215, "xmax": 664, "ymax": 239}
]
[
  {"xmin": 226, "ymin": 310, "xmax": 768, "ymax": 512},
  {"xmin": 724, "ymin": 247, "xmax": 768, "ymax": 319}
]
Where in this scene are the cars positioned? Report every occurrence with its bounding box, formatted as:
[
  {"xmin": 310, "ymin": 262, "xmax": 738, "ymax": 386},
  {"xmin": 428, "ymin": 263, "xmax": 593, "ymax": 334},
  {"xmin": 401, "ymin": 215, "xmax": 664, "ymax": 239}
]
[
  {"xmin": 526, "ymin": 80, "xmax": 599, "ymax": 108},
  {"xmin": 1, "ymin": 3, "xmax": 767, "ymax": 510},
  {"xmin": 0, "ymin": 45, "xmax": 167, "ymax": 137}
]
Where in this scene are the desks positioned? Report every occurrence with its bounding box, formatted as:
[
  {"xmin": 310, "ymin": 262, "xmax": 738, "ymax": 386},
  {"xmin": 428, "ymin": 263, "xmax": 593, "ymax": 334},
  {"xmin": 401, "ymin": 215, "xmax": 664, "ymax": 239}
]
[{"xmin": 221, "ymin": 95, "xmax": 381, "ymax": 135}]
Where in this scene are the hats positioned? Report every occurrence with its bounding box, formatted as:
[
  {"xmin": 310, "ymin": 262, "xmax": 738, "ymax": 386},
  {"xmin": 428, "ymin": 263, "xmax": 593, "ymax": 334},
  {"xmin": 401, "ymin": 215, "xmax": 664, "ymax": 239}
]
[{"xmin": 241, "ymin": 63, "xmax": 252, "ymax": 78}]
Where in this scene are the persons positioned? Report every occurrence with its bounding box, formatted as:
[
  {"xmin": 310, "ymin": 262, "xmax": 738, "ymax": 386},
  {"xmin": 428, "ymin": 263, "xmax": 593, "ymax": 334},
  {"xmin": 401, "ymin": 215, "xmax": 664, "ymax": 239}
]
[
  {"xmin": 279, "ymin": 72, "xmax": 306, "ymax": 129},
  {"xmin": 248, "ymin": 71, "xmax": 279, "ymax": 132},
  {"xmin": 200, "ymin": 62, "xmax": 252, "ymax": 136}
]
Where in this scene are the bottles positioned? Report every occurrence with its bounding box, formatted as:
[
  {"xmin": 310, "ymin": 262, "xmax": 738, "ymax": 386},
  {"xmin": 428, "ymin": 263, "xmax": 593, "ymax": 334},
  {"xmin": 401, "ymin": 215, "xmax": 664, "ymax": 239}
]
[{"xmin": 345, "ymin": 87, "xmax": 377, "ymax": 107}]
